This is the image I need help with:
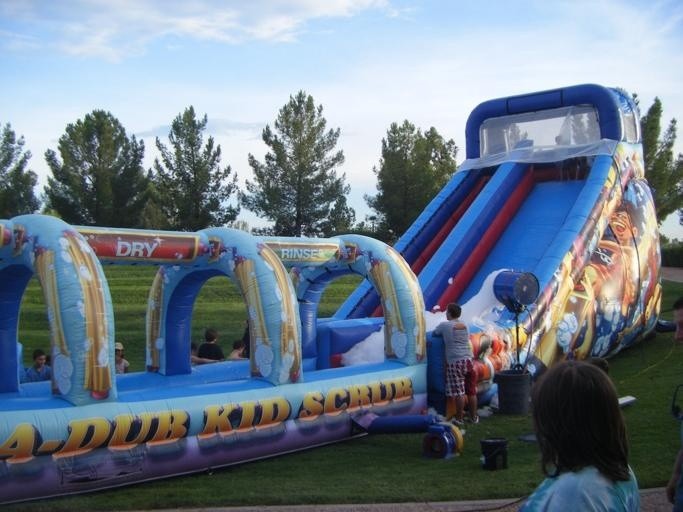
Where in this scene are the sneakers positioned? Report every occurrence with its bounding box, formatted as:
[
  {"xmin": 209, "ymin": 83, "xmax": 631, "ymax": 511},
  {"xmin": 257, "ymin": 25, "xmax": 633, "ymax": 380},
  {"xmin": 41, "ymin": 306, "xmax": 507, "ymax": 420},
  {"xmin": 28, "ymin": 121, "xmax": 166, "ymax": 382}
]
[{"xmin": 454, "ymin": 417, "xmax": 478, "ymax": 426}]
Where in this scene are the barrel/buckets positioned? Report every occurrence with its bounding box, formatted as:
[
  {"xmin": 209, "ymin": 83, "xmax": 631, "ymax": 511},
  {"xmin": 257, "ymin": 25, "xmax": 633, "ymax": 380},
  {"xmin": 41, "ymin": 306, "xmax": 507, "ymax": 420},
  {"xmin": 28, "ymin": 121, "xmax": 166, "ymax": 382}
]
[
  {"xmin": 493, "ymin": 370, "xmax": 530, "ymax": 416},
  {"xmin": 480, "ymin": 437, "xmax": 508, "ymax": 470}
]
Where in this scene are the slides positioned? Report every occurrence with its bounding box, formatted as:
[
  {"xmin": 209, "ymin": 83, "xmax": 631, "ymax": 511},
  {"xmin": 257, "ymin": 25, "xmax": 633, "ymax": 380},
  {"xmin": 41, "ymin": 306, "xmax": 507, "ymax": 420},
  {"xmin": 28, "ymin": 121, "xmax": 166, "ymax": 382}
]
[
  {"xmin": 417, "ymin": 83, "xmax": 664, "ymax": 416},
  {"xmin": 317, "ymin": 91, "xmax": 533, "ymax": 367}
]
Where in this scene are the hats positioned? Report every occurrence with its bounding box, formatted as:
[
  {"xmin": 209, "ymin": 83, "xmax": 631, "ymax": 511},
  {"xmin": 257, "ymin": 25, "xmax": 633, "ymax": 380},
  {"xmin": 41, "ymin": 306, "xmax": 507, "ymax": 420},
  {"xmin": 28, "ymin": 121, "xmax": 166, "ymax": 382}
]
[{"xmin": 115, "ymin": 342, "xmax": 124, "ymax": 350}]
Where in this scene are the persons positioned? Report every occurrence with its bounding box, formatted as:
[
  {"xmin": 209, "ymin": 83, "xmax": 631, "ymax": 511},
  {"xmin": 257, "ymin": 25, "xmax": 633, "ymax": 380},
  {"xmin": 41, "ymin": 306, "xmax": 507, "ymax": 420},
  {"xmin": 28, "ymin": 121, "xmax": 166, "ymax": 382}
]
[
  {"xmin": 44, "ymin": 354, "xmax": 51, "ymax": 364},
  {"xmin": 198, "ymin": 327, "xmax": 224, "ymax": 364},
  {"xmin": 242, "ymin": 321, "xmax": 250, "ymax": 357},
  {"xmin": 114, "ymin": 342, "xmax": 129, "ymax": 373},
  {"xmin": 431, "ymin": 302, "xmax": 480, "ymax": 425},
  {"xmin": 24, "ymin": 349, "xmax": 52, "ymax": 382},
  {"xmin": 666, "ymin": 296, "xmax": 682, "ymax": 512},
  {"xmin": 517, "ymin": 360, "xmax": 641, "ymax": 511},
  {"xmin": 226, "ymin": 339, "xmax": 248, "ymax": 360},
  {"xmin": 590, "ymin": 356, "xmax": 609, "ymax": 375},
  {"xmin": 189, "ymin": 342, "xmax": 221, "ymax": 365},
  {"xmin": 553, "ymin": 135, "xmax": 594, "ymax": 181}
]
